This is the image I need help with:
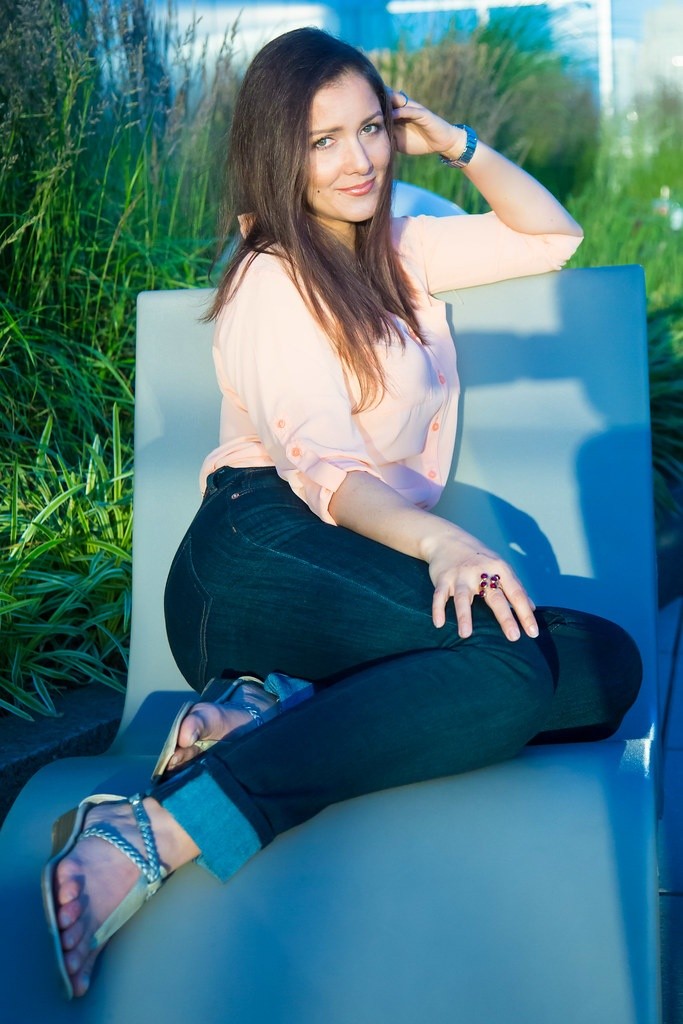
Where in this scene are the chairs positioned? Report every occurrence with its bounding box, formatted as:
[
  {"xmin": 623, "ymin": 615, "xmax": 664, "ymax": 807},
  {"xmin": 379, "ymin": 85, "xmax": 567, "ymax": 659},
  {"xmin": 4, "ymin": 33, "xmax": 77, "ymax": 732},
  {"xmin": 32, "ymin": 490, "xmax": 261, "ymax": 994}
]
[{"xmin": 0, "ymin": 264, "xmax": 665, "ymax": 1024}]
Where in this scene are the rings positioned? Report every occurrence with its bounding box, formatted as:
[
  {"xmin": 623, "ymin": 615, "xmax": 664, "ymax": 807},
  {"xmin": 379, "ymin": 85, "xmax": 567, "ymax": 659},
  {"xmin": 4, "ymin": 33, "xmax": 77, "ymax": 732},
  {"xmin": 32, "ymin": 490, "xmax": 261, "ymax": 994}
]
[
  {"xmin": 398, "ymin": 90, "xmax": 408, "ymax": 108},
  {"xmin": 479, "ymin": 573, "xmax": 504, "ymax": 597}
]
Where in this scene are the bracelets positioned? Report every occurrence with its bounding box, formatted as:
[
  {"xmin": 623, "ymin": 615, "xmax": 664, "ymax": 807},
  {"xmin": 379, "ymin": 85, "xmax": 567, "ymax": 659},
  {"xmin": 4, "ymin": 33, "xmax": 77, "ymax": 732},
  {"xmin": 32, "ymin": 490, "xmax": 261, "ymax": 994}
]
[{"xmin": 440, "ymin": 124, "xmax": 478, "ymax": 169}]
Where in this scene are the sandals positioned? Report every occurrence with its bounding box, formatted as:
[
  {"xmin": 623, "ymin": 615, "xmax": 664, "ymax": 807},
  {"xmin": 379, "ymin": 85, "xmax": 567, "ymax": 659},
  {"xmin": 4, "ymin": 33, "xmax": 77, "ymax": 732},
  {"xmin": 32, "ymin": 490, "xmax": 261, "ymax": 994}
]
[
  {"xmin": 148, "ymin": 673, "xmax": 285, "ymax": 787},
  {"xmin": 39, "ymin": 789, "xmax": 173, "ymax": 1004}
]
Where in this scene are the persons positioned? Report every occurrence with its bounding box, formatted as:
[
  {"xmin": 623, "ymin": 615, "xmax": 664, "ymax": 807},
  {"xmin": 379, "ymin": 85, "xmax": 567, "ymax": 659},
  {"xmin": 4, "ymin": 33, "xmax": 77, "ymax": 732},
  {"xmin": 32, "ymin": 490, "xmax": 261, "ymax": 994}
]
[{"xmin": 42, "ymin": 26, "xmax": 646, "ymax": 998}]
